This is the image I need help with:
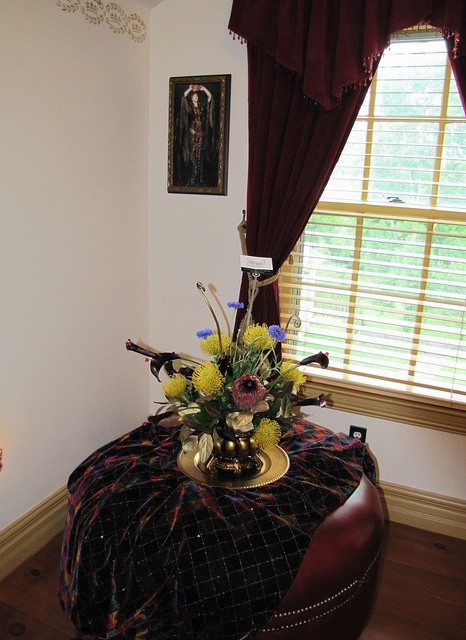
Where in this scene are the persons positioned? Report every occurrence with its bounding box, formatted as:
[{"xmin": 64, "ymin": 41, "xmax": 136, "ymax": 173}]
[{"xmin": 179, "ymin": 84, "xmax": 215, "ymax": 187}]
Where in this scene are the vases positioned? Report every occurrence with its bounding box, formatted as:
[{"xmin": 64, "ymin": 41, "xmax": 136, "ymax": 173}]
[{"xmin": 206, "ymin": 423, "xmax": 263, "ymax": 478}]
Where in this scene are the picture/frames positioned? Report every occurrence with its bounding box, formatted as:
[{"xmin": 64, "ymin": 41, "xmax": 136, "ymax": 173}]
[{"xmin": 167, "ymin": 75, "xmax": 232, "ymax": 195}]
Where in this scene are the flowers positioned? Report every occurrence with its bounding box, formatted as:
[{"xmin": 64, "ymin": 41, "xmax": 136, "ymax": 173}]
[{"xmin": 126, "ymin": 282, "xmax": 329, "ymax": 475}]
